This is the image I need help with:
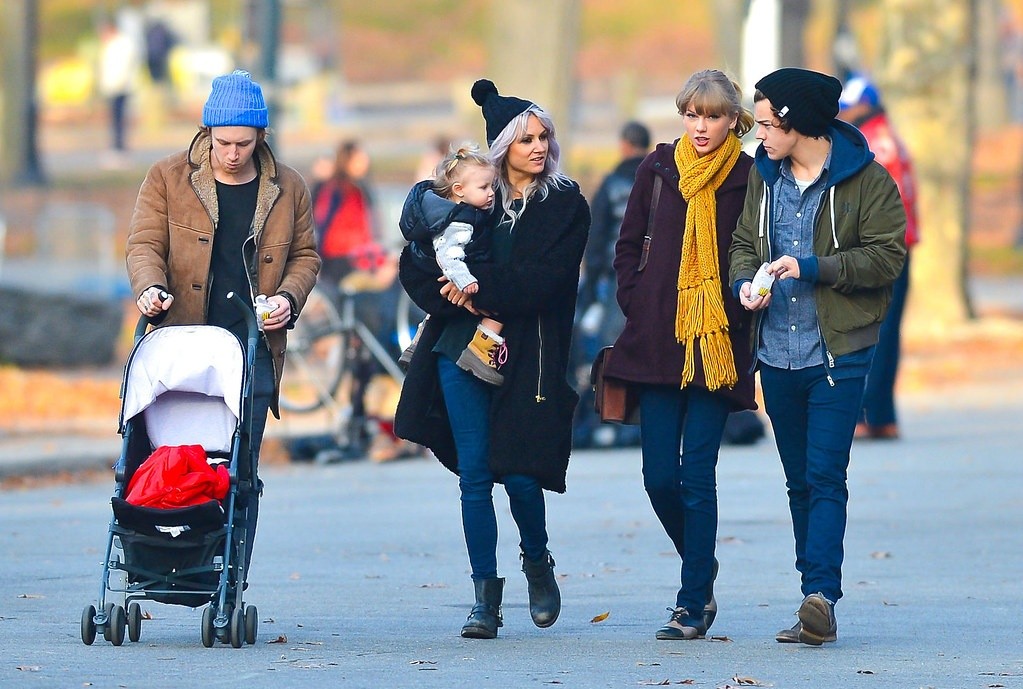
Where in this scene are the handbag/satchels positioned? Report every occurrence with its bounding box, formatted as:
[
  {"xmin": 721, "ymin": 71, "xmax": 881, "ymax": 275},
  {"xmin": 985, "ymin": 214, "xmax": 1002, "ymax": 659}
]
[{"xmin": 590, "ymin": 346, "xmax": 641, "ymax": 426}]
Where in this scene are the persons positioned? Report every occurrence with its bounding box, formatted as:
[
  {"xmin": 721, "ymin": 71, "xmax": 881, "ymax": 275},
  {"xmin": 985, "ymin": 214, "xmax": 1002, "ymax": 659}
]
[
  {"xmin": 583, "ymin": 123, "xmax": 653, "ymax": 345},
  {"xmin": 394, "ymin": 79, "xmax": 591, "ymax": 639},
  {"xmin": 398, "ymin": 143, "xmax": 508, "ymax": 386},
  {"xmin": 98, "ymin": 20, "xmax": 138, "ymax": 151},
  {"xmin": 729, "ymin": 68, "xmax": 907, "ymax": 645},
  {"xmin": 600, "ymin": 69, "xmax": 758, "ymax": 640},
  {"xmin": 838, "ymin": 77, "xmax": 921, "ymax": 438},
  {"xmin": 125, "ymin": 68, "xmax": 321, "ymax": 591},
  {"xmin": 309, "ymin": 139, "xmax": 386, "ymax": 269}
]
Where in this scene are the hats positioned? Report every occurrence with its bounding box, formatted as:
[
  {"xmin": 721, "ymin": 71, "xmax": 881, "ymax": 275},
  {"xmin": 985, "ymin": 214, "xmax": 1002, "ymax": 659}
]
[
  {"xmin": 202, "ymin": 69, "xmax": 269, "ymax": 129},
  {"xmin": 838, "ymin": 69, "xmax": 878, "ymax": 110},
  {"xmin": 755, "ymin": 67, "xmax": 843, "ymax": 137},
  {"xmin": 471, "ymin": 79, "xmax": 535, "ymax": 149}
]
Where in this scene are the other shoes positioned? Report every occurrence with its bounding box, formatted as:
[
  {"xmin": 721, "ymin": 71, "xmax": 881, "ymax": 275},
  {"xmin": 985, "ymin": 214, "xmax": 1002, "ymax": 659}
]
[
  {"xmin": 775, "ymin": 617, "xmax": 838, "ymax": 643},
  {"xmin": 793, "ymin": 591, "xmax": 835, "ymax": 646},
  {"xmin": 854, "ymin": 424, "xmax": 897, "ymax": 438}
]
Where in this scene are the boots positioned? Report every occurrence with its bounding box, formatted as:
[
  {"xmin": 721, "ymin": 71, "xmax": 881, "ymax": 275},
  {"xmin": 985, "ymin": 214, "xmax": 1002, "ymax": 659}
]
[
  {"xmin": 455, "ymin": 323, "xmax": 508, "ymax": 387},
  {"xmin": 398, "ymin": 319, "xmax": 426, "ymax": 377},
  {"xmin": 461, "ymin": 577, "xmax": 505, "ymax": 638},
  {"xmin": 519, "ymin": 550, "xmax": 561, "ymax": 628}
]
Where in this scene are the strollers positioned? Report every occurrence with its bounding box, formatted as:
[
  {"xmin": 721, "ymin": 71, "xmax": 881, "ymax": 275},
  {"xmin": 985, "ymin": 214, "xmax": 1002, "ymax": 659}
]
[{"xmin": 81, "ymin": 291, "xmax": 265, "ymax": 649}]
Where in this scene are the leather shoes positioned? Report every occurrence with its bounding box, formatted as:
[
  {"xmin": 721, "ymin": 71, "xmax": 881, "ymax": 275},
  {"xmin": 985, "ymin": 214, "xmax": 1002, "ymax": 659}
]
[
  {"xmin": 656, "ymin": 606, "xmax": 707, "ymax": 641},
  {"xmin": 703, "ymin": 556, "xmax": 720, "ymax": 630}
]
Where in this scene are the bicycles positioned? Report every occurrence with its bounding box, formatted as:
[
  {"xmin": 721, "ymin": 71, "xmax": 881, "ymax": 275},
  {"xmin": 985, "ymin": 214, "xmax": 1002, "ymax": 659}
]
[{"xmin": 263, "ymin": 273, "xmax": 350, "ymax": 415}]
